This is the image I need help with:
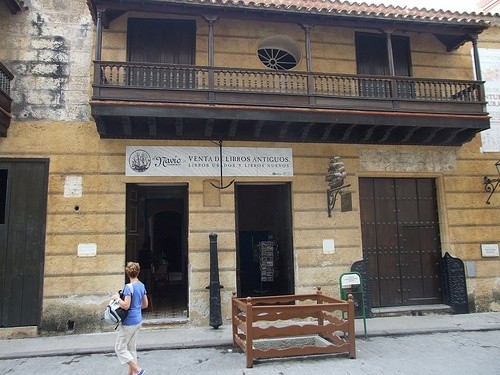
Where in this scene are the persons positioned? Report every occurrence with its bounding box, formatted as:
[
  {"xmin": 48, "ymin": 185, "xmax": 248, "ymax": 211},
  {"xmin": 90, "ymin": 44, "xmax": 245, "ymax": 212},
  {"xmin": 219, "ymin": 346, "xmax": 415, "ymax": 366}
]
[{"xmin": 114, "ymin": 262, "xmax": 149, "ymax": 375}]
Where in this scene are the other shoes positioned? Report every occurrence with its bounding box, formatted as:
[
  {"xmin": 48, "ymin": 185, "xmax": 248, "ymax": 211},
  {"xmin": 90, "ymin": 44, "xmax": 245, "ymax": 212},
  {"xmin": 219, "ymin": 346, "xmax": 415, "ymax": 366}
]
[{"xmin": 135, "ymin": 368, "xmax": 145, "ymax": 375}]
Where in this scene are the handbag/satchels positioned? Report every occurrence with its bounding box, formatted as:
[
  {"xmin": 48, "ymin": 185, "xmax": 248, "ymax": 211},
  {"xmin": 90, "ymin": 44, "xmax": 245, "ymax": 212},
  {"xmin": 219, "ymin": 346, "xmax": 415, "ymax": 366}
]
[{"xmin": 101, "ymin": 284, "xmax": 133, "ymax": 330}]
